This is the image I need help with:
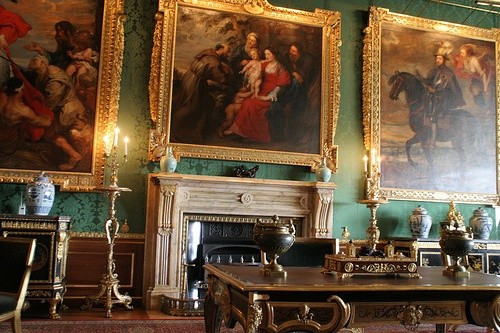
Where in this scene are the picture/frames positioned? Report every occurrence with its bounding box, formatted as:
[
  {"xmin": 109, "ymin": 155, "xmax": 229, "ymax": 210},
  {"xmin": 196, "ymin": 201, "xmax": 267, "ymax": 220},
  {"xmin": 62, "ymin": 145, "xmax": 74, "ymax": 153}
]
[
  {"xmin": 149, "ymin": 0, "xmax": 339, "ymax": 173},
  {"xmin": 0, "ymin": 0, "xmax": 124, "ymax": 192},
  {"xmin": 363, "ymin": 6, "xmax": 500, "ymax": 208}
]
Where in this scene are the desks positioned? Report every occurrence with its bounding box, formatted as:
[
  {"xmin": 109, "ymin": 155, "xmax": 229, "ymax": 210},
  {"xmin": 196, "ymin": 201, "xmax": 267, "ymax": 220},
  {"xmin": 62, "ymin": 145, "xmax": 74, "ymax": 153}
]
[{"xmin": 203, "ymin": 263, "xmax": 500, "ymax": 333}]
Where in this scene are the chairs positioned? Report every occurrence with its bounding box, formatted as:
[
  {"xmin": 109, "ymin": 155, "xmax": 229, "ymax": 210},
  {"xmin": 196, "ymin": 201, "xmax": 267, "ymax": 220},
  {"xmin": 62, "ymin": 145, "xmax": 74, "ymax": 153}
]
[
  {"xmin": 278, "ymin": 237, "xmax": 338, "ymax": 268},
  {"xmin": 0, "ymin": 239, "xmax": 38, "ymax": 333}
]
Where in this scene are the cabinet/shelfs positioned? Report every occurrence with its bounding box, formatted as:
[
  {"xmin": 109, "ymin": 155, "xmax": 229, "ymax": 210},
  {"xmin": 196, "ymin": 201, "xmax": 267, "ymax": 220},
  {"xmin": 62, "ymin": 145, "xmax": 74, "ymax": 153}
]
[
  {"xmin": 0, "ymin": 213, "xmax": 72, "ymax": 318},
  {"xmin": 392, "ymin": 238, "xmax": 500, "ymax": 273}
]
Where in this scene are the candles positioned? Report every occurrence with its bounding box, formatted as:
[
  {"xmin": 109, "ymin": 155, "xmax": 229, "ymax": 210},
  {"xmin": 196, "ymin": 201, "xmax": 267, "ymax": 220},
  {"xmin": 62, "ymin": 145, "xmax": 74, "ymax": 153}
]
[
  {"xmin": 370, "ymin": 148, "xmax": 376, "ymax": 164},
  {"xmin": 124, "ymin": 137, "xmax": 130, "ymax": 156},
  {"xmin": 114, "ymin": 127, "xmax": 120, "ymax": 146},
  {"xmin": 104, "ymin": 135, "xmax": 110, "ymax": 152},
  {"xmin": 367, "ymin": 160, "xmax": 372, "ymax": 178},
  {"xmin": 376, "ymin": 158, "xmax": 381, "ymax": 173},
  {"xmin": 363, "ymin": 155, "xmax": 368, "ymax": 172}
]
